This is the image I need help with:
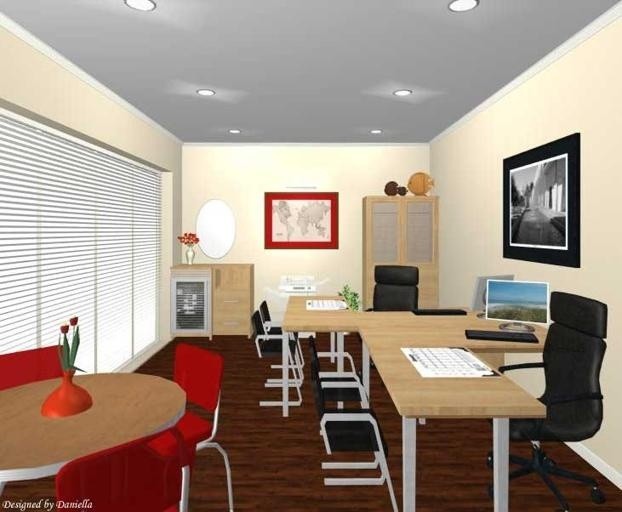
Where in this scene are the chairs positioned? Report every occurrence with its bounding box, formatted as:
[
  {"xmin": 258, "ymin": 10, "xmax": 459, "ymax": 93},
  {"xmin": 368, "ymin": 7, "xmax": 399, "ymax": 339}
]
[
  {"xmin": 56, "ymin": 429, "xmax": 182, "ymax": 512},
  {"xmin": 488, "ymin": 291, "xmax": 608, "ymax": 512},
  {"xmin": 0, "ymin": 345, "xmax": 66, "ymax": 497},
  {"xmin": 174, "ymin": 343, "xmax": 234, "ymax": 512},
  {"xmin": 252, "ymin": 301, "xmax": 388, "ymax": 486},
  {"xmin": 359, "ymin": 265, "xmax": 419, "ymax": 368}
]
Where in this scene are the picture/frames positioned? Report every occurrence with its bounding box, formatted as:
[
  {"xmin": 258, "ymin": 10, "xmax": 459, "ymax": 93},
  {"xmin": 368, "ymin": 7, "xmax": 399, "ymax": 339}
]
[
  {"xmin": 264, "ymin": 192, "xmax": 338, "ymax": 249},
  {"xmin": 503, "ymin": 133, "xmax": 580, "ymax": 269}
]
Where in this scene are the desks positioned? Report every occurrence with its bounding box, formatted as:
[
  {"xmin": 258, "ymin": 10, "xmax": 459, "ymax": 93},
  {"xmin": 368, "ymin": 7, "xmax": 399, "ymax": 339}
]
[
  {"xmin": 283, "ymin": 295, "xmax": 426, "ymax": 424},
  {"xmin": 357, "ymin": 316, "xmax": 549, "ymax": 512},
  {"xmin": 0, "ymin": 373, "xmax": 188, "ymax": 482}
]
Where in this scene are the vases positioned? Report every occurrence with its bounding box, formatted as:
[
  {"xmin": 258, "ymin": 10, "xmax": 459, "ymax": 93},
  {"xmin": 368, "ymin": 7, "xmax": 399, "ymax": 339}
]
[
  {"xmin": 186, "ymin": 245, "xmax": 195, "ymax": 265},
  {"xmin": 41, "ymin": 371, "xmax": 92, "ymax": 417}
]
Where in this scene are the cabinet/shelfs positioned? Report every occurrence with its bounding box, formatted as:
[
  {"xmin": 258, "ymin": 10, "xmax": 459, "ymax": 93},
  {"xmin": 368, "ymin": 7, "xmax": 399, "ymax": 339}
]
[
  {"xmin": 363, "ymin": 196, "xmax": 439, "ymax": 309},
  {"xmin": 170, "ymin": 264, "xmax": 254, "ymax": 341}
]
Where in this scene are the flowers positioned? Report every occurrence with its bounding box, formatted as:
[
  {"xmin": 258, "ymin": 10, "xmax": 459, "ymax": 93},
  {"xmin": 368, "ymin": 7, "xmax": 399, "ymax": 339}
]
[
  {"xmin": 58, "ymin": 318, "xmax": 84, "ymax": 373},
  {"xmin": 177, "ymin": 233, "xmax": 199, "ymax": 245}
]
